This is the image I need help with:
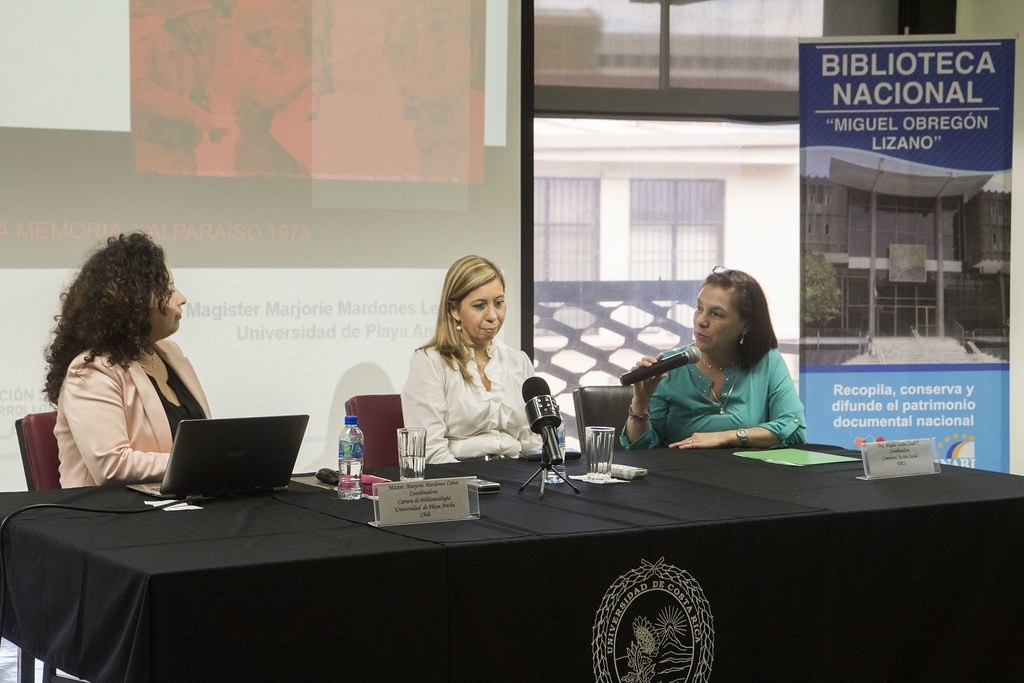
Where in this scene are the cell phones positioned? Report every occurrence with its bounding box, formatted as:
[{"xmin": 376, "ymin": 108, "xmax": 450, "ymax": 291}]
[{"xmin": 467, "ymin": 479, "xmax": 500, "ymax": 495}]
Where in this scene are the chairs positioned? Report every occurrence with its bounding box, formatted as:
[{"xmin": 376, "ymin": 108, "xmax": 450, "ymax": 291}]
[
  {"xmin": 345, "ymin": 395, "xmax": 407, "ymax": 470},
  {"xmin": 573, "ymin": 386, "xmax": 635, "ymax": 453},
  {"xmin": 16, "ymin": 411, "xmax": 93, "ymax": 683}
]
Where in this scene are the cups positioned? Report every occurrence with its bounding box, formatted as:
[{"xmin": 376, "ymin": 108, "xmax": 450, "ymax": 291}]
[
  {"xmin": 396, "ymin": 425, "xmax": 428, "ymax": 482},
  {"xmin": 585, "ymin": 425, "xmax": 616, "ymax": 485}
]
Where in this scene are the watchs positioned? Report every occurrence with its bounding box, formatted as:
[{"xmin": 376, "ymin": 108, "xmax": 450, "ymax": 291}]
[{"xmin": 737, "ymin": 429, "xmax": 748, "ymax": 446}]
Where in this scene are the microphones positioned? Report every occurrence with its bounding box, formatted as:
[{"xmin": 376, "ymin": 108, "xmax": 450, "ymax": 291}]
[
  {"xmin": 522, "ymin": 377, "xmax": 563, "ymax": 465},
  {"xmin": 620, "ymin": 346, "xmax": 702, "ymax": 386}
]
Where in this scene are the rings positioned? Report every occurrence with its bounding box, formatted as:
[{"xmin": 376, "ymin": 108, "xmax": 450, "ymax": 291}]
[{"xmin": 690, "ymin": 439, "xmax": 693, "ymax": 443}]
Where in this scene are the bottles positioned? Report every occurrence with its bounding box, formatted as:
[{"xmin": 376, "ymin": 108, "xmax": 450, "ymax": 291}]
[
  {"xmin": 337, "ymin": 413, "xmax": 365, "ymax": 502},
  {"xmin": 541, "ymin": 410, "xmax": 567, "ymax": 485}
]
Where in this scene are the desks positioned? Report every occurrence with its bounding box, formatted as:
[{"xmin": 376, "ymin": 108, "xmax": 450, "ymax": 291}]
[{"xmin": 0, "ymin": 444, "xmax": 1024, "ymax": 683}]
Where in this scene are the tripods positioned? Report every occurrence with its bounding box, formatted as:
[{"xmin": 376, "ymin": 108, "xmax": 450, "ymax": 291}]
[{"xmin": 519, "ymin": 426, "xmax": 580, "ymax": 498}]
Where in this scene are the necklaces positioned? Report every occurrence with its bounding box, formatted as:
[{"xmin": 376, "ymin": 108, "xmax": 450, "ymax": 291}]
[
  {"xmin": 139, "ymin": 357, "xmax": 155, "ymax": 371},
  {"xmin": 703, "ymin": 359, "xmax": 741, "ymax": 415}
]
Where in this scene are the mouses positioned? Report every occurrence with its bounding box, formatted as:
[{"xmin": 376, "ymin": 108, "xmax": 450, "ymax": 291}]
[{"xmin": 316, "ymin": 468, "xmax": 339, "ymax": 485}]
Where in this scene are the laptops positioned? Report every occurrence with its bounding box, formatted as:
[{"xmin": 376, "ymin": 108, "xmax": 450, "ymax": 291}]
[{"xmin": 126, "ymin": 415, "xmax": 310, "ymax": 498}]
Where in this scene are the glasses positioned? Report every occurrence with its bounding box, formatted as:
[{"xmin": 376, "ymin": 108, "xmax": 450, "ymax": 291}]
[{"xmin": 712, "ymin": 266, "xmax": 753, "ymax": 289}]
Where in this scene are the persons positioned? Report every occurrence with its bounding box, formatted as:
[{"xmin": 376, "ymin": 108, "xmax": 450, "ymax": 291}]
[
  {"xmin": 42, "ymin": 233, "xmax": 212, "ymax": 489},
  {"xmin": 618, "ymin": 270, "xmax": 807, "ymax": 449},
  {"xmin": 401, "ymin": 255, "xmax": 542, "ymax": 464}
]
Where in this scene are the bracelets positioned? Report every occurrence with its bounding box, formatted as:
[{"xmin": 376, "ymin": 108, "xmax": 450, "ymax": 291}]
[{"xmin": 629, "ymin": 405, "xmax": 650, "ymax": 422}]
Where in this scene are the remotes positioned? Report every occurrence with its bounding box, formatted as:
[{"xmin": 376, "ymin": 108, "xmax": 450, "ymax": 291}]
[{"xmin": 588, "ymin": 462, "xmax": 648, "ymax": 480}]
